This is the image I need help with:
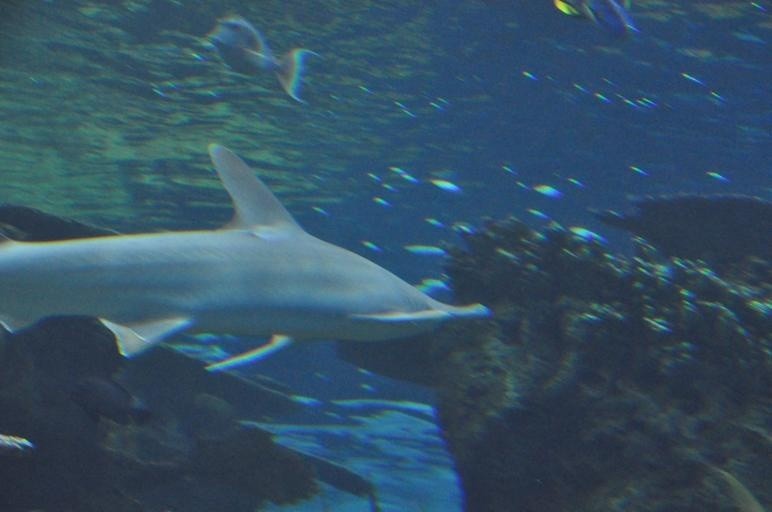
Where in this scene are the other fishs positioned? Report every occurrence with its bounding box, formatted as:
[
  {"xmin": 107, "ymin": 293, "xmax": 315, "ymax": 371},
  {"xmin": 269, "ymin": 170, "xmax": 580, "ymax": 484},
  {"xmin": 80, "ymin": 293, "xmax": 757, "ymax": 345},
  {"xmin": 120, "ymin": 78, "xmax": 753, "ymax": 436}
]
[{"xmin": 0, "ymin": 0, "xmax": 772, "ymax": 511}]
